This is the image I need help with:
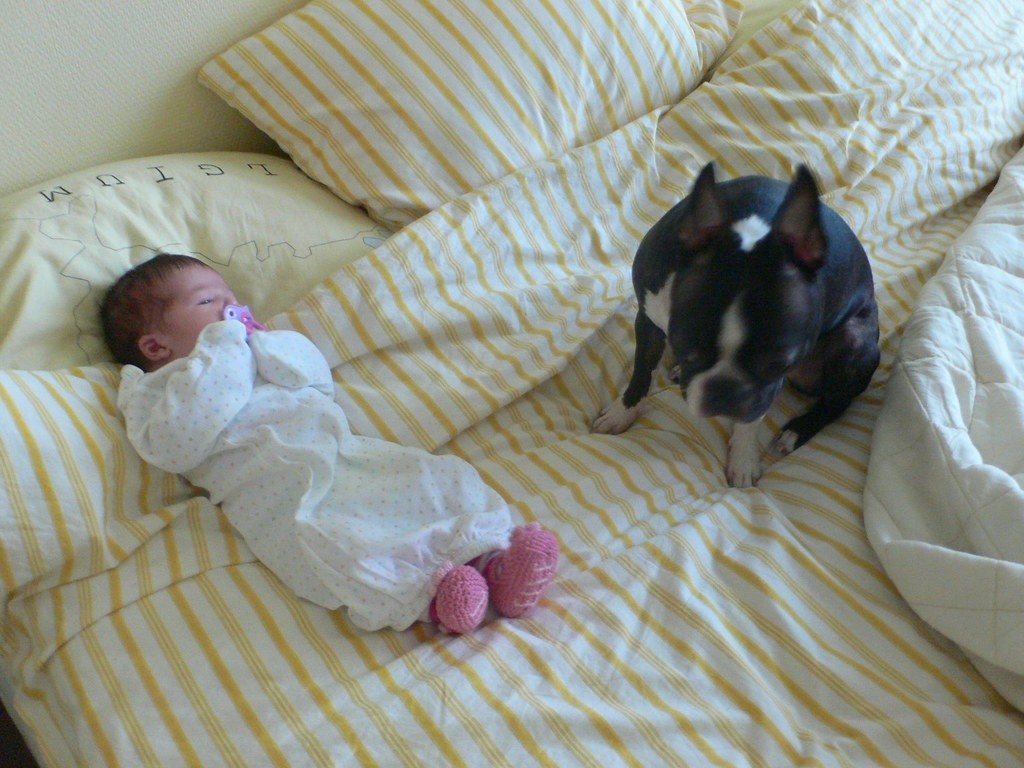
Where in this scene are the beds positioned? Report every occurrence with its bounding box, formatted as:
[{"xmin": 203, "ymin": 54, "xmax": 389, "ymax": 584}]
[{"xmin": 0, "ymin": 0, "xmax": 1023, "ymax": 768}]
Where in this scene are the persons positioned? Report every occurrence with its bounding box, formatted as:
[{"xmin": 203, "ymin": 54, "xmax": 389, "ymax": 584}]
[{"xmin": 101, "ymin": 253, "xmax": 560, "ymax": 633}]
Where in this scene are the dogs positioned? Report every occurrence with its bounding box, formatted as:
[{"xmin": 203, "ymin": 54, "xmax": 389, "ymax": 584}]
[{"xmin": 591, "ymin": 160, "xmax": 882, "ymax": 488}]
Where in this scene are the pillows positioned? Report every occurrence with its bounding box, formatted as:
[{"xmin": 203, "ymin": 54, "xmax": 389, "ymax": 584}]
[
  {"xmin": 0, "ymin": 151, "xmax": 395, "ymax": 371},
  {"xmin": 195, "ymin": 0, "xmax": 743, "ymax": 233}
]
